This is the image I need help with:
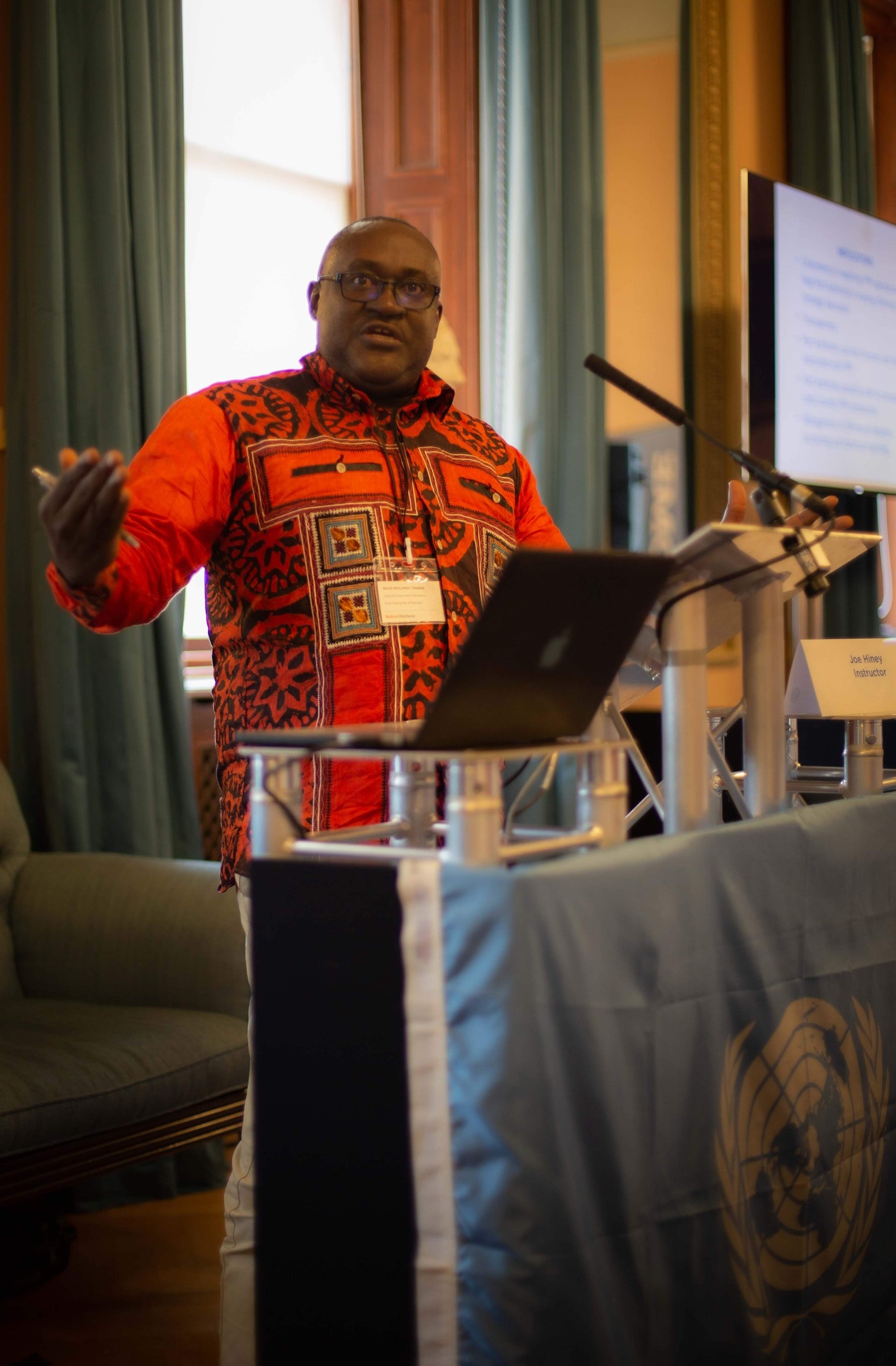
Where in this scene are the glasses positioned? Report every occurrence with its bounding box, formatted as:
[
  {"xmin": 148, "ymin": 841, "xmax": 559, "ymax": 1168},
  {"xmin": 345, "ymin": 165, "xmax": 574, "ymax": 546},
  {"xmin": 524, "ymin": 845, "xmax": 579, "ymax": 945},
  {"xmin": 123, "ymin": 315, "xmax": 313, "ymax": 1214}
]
[{"xmin": 317, "ymin": 272, "xmax": 440, "ymax": 311}]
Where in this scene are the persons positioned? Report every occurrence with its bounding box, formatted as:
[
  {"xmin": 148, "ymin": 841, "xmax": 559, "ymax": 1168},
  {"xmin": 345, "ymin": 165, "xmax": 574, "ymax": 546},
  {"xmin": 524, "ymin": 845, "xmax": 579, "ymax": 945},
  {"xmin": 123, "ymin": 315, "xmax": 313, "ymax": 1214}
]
[{"xmin": 40, "ymin": 218, "xmax": 574, "ymax": 1366}]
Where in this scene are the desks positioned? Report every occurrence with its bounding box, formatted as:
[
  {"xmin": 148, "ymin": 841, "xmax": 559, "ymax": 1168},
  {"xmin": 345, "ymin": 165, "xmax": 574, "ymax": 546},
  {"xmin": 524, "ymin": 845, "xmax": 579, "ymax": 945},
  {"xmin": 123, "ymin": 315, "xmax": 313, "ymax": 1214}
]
[{"xmin": 235, "ymin": 740, "xmax": 633, "ymax": 869}]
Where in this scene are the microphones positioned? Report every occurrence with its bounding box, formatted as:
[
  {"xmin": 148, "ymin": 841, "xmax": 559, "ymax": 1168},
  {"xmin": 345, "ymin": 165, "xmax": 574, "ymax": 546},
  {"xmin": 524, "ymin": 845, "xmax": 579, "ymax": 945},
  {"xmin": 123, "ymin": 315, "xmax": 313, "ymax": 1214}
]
[{"xmin": 583, "ymin": 353, "xmax": 837, "ymax": 521}]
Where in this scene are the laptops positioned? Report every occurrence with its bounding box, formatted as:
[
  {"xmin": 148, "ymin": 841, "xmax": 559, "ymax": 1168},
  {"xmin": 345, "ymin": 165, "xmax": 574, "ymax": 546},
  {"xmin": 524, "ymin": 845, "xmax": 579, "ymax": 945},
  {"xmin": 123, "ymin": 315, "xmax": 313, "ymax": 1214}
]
[{"xmin": 235, "ymin": 549, "xmax": 678, "ymax": 749}]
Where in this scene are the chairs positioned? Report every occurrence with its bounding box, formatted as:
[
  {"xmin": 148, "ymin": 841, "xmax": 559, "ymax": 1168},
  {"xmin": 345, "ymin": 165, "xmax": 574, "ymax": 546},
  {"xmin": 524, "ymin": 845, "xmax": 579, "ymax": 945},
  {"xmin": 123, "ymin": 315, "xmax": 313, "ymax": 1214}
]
[{"xmin": 0, "ymin": 759, "xmax": 249, "ymax": 1296}]
